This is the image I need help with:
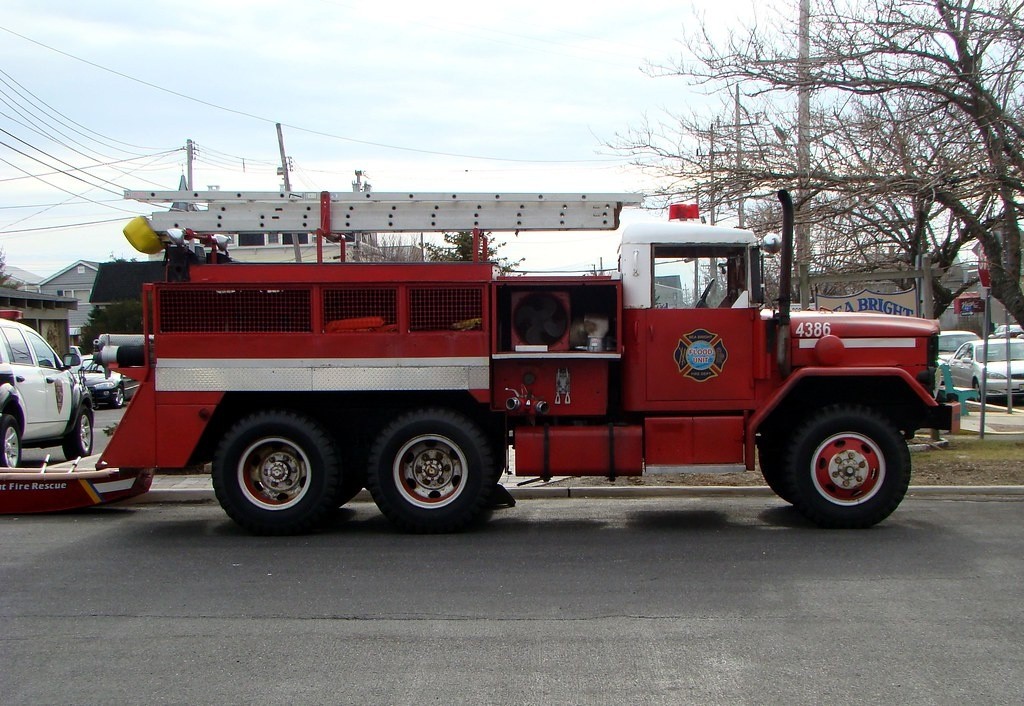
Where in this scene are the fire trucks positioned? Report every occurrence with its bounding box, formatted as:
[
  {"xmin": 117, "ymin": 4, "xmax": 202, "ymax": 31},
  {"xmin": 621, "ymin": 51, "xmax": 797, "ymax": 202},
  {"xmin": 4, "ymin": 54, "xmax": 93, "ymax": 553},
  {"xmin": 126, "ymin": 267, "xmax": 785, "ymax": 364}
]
[{"xmin": 95, "ymin": 187, "xmax": 963, "ymax": 534}]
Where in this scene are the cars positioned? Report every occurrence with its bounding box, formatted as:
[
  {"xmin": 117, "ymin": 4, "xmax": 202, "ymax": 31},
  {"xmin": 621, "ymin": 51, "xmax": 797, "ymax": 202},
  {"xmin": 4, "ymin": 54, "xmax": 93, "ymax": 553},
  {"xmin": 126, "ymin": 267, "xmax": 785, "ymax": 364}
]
[
  {"xmin": 988, "ymin": 323, "xmax": 1024, "ymax": 339},
  {"xmin": 938, "ymin": 330, "xmax": 985, "ymax": 384},
  {"xmin": 946, "ymin": 338, "xmax": 1024, "ymax": 401},
  {"xmin": 66, "ymin": 354, "xmax": 140, "ymax": 409}
]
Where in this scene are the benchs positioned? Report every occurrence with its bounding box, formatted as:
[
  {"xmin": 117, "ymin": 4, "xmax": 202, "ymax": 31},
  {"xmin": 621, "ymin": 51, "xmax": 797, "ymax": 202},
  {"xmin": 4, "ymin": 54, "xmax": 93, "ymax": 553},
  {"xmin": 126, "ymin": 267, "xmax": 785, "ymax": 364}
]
[{"xmin": 937, "ymin": 359, "xmax": 978, "ymax": 416}]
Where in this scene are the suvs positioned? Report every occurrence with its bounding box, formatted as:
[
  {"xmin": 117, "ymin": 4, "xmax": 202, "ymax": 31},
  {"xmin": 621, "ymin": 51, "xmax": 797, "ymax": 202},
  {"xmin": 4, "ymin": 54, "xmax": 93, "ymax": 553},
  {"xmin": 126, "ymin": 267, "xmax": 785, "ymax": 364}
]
[{"xmin": 0, "ymin": 309, "xmax": 96, "ymax": 468}]
